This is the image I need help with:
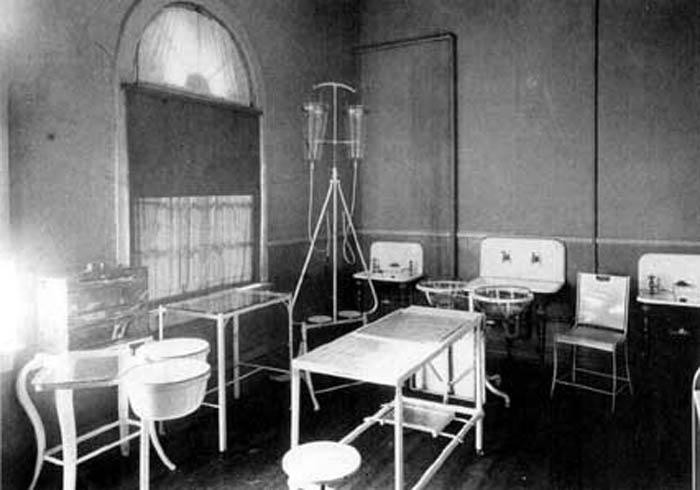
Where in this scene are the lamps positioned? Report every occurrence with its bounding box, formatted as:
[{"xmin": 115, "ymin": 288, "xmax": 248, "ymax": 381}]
[{"xmin": 286, "ymin": 77, "xmax": 379, "ymax": 412}]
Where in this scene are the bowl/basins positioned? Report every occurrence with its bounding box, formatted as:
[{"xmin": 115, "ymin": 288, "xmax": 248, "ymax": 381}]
[{"xmin": 120, "ymin": 335, "xmax": 212, "ymax": 425}]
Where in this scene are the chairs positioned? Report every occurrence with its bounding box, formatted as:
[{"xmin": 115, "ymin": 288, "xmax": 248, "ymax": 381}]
[
  {"xmin": 547, "ymin": 270, "xmax": 635, "ymax": 416},
  {"xmin": 351, "ymin": 234, "xmax": 426, "ymax": 322}
]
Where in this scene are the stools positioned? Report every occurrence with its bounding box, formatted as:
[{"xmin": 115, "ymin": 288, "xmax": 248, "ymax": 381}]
[{"xmin": 279, "ymin": 440, "xmax": 364, "ymax": 490}]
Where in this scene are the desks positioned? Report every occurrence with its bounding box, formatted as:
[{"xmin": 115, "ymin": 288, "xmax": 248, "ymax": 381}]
[
  {"xmin": 156, "ymin": 284, "xmax": 301, "ymax": 455},
  {"xmin": 465, "ymin": 234, "xmax": 699, "ymax": 369},
  {"xmin": 14, "ymin": 333, "xmax": 178, "ymax": 490},
  {"xmin": 287, "ymin": 302, "xmax": 492, "ymax": 490}
]
